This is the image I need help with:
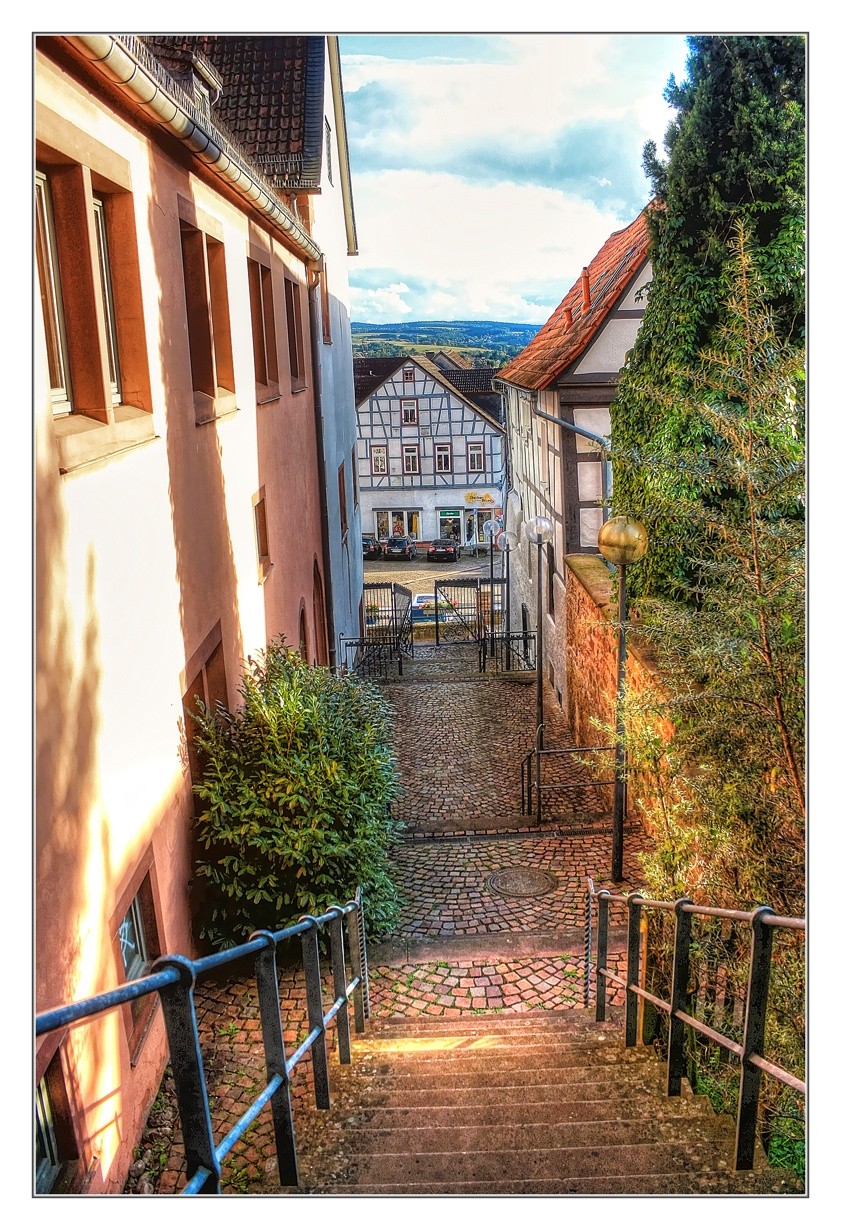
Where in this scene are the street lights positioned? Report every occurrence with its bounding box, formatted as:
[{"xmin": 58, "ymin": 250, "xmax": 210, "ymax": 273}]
[
  {"xmin": 497, "ymin": 531, "xmax": 519, "ymax": 671},
  {"xmin": 597, "ymin": 514, "xmax": 648, "ymax": 882},
  {"xmin": 525, "ymin": 516, "xmax": 554, "ymax": 751},
  {"xmin": 482, "ymin": 519, "xmax": 500, "ymax": 657}
]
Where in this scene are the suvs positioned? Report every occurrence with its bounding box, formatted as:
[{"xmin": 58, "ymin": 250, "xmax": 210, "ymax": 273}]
[{"xmin": 383, "ymin": 535, "xmax": 417, "ymax": 561}]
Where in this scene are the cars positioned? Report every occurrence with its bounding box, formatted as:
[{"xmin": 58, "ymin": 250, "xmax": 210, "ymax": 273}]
[
  {"xmin": 408, "ymin": 593, "xmax": 448, "ymax": 622},
  {"xmin": 362, "ymin": 535, "xmax": 383, "ymax": 561},
  {"xmin": 427, "ymin": 539, "xmax": 461, "ymax": 562}
]
